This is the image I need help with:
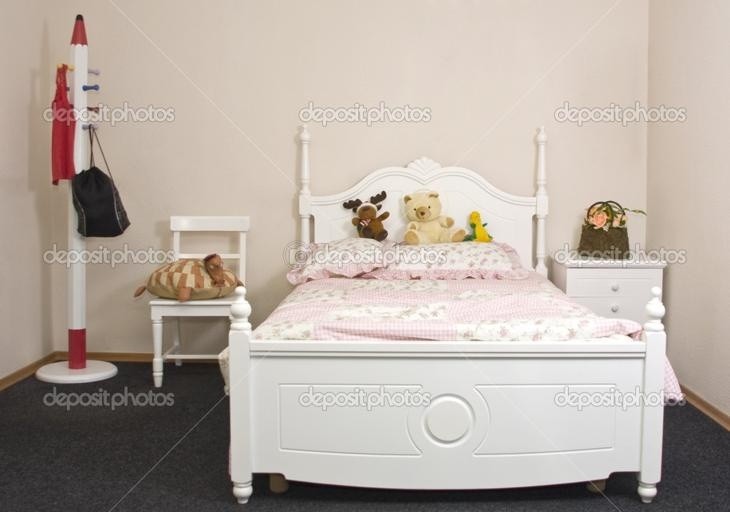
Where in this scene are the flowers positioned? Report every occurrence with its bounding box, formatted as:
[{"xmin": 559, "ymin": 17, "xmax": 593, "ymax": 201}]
[{"xmin": 581, "ymin": 200, "xmax": 646, "ymax": 230}]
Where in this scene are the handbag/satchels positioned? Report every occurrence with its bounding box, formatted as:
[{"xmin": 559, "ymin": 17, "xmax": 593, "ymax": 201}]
[{"xmin": 71, "ymin": 166, "xmax": 131, "ymax": 237}]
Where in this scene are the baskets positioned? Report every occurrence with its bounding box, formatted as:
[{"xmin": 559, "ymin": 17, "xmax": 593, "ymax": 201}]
[{"xmin": 579, "ymin": 200, "xmax": 630, "ymax": 260}]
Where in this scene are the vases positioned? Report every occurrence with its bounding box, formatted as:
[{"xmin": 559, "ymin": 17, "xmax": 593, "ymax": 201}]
[{"xmin": 578, "ymin": 225, "xmax": 630, "ymax": 258}]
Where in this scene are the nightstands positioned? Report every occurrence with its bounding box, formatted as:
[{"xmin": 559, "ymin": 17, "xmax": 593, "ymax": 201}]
[{"xmin": 553, "ymin": 259, "xmax": 664, "ymax": 328}]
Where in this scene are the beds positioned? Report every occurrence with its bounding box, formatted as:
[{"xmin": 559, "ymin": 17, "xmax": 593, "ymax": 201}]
[{"xmin": 228, "ymin": 121, "xmax": 667, "ymax": 504}]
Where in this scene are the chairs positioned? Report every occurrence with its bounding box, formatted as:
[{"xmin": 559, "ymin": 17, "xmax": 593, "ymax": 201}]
[{"xmin": 149, "ymin": 216, "xmax": 249, "ymax": 398}]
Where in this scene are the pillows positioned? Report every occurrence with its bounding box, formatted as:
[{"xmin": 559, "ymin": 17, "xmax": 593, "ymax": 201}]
[
  {"xmin": 285, "ymin": 235, "xmax": 386, "ymax": 286},
  {"xmin": 361, "ymin": 241, "xmax": 528, "ymax": 279}
]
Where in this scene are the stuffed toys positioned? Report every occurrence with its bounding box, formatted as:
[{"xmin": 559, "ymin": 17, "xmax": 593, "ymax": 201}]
[
  {"xmin": 401, "ymin": 192, "xmax": 466, "ymax": 244},
  {"xmin": 133, "ymin": 254, "xmax": 244, "ymax": 301},
  {"xmin": 465, "ymin": 209, "xmax": 494, "ymax": 243},
  {"xmin": 342, "ymin": 190, "xmax": 390, "ymax": 242}
]
